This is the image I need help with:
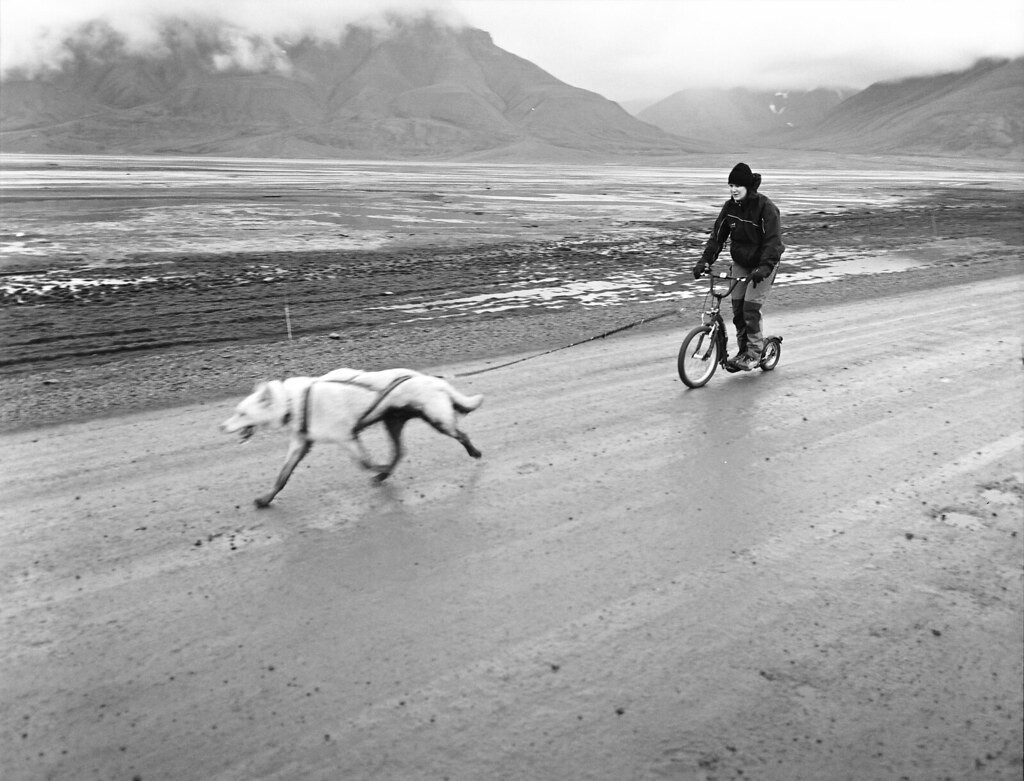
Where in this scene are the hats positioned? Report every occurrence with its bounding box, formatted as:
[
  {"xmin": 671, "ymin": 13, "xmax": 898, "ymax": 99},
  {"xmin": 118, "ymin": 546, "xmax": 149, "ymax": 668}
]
[{"xmin": 728, "ymin": 163, "xmax": 753, "ymax": 186}]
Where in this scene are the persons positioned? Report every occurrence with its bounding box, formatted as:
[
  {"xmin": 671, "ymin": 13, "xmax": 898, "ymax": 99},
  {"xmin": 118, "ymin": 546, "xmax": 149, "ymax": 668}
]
[{"xmin": 694, "ymin": 163, "xmax": 786, "ymax": 369}]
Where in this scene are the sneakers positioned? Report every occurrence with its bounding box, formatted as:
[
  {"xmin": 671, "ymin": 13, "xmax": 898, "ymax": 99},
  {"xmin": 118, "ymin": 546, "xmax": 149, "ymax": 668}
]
[
  {"xmin": 728, "ymin": 351, "xmax": 748, "ymax": 366},
  {"xmin": 737, "ymin": 351, "xmax": 761, "ymax": 370}
]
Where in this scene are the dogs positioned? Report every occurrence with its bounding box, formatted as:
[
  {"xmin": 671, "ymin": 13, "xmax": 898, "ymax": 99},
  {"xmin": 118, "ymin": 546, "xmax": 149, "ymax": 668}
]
[{"xmin": 219, "ymin": 367, "xmax": 483, "ymax": 509}]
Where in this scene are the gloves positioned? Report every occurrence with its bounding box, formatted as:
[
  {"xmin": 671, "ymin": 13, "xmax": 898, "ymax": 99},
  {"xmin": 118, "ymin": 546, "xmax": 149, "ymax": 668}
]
[
  {"xmin": 694, "ymin": 260, "xmax": 712, "ymax": 279},
  {"xmin": 751, "ymin": 269, "xmax": 763, "ymax": 289}
]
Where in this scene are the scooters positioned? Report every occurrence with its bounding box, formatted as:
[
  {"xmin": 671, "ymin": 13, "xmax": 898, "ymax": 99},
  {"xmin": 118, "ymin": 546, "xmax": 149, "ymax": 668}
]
[{"xmin": 675, "ymin": 262, "xmax": 783, "ymax": 389}]
